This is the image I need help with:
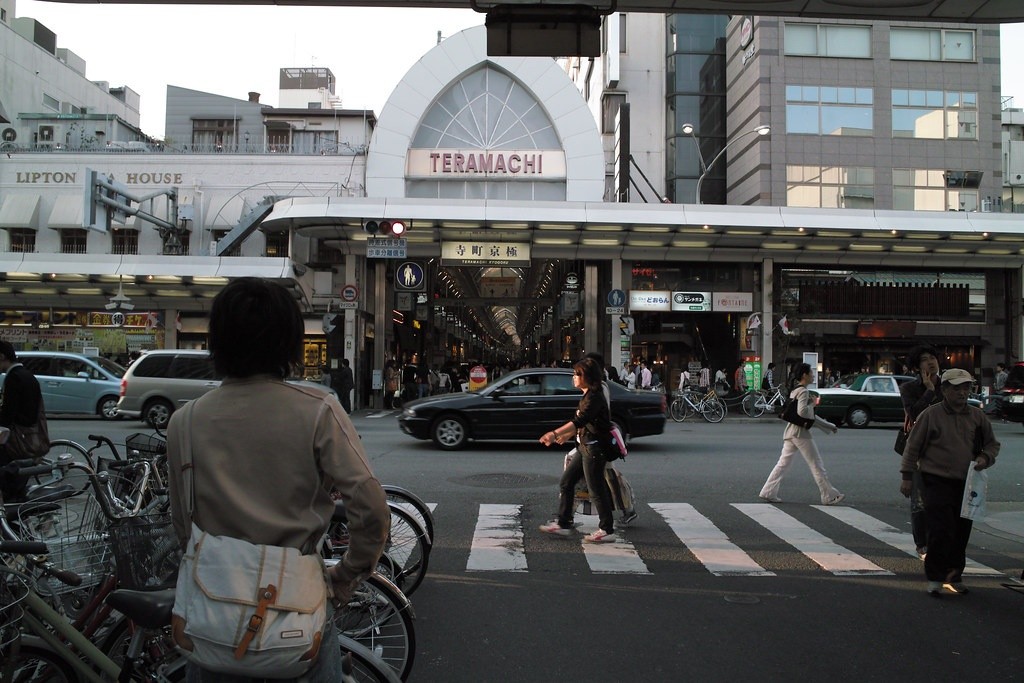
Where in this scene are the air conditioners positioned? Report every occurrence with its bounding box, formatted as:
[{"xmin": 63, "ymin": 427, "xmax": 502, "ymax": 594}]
[
  {"xmin": 0, "ymin": 127, "xmax": 34, "ymax": 151},
  {"xmin": 38, "ymin": 124, "xmax": 62, "ymax": 145}
]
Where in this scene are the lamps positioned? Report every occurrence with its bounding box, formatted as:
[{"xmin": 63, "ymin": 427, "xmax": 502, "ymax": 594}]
[
  {"xmin": 682, "ymin": 123, "xmax": 771, "ymax": 204},
  {"xmin": 244, "ymin": 131, "xmax": 250, "ymax": 140}
]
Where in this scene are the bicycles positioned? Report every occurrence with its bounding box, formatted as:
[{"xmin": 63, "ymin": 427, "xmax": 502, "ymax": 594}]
[
  {"xmin": 742, "ymin": 384, "xmax": 785, "ymax": 419},
  {"xmin": 0, "ymin": 410, "xmax": 434, "ymax": 683},
  {"xmin": 668, "ymin": 383, "xmax": 752, "ymax": 423}
]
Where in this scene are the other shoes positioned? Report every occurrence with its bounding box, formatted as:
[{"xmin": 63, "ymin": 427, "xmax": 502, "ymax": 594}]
[
  {"xmin": 918, "ymin": 553, "xmax": 926, "ymax": 560},
  {"xmin": 759, "ymin": 495, "xmax": 781, "ymax": 503},
  {"xmin": 548, "ymin": 519, "xmax": 574, "ymax": 526},
  {"xmin": 822, "ymin": 494, "xmax": 844, "ymax": 505},
  {"xmin": 942, "ymin": 582, "xmax": 969, "ymax": 593},
  {"xmin": 621, "ymin": 511, "xmax": 637, "ymax": 524},
  {"xmin": 926, "ymin": 581, "xmax": 941, "ymax": 596},
  {"xmin": 540, "ymin": 524, "xmax": 569, "ymax": 536},
  {"xmin": 585, "ymin": 530, "xmax": 616, "ymax": 543}
]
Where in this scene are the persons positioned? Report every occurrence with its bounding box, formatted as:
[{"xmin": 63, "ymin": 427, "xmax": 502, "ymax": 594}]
[
  {"xmin": 537, "ymin": 357, "xmax": 638, "ymax": 539},
  {"xmin": 0, "ymin": 322, "xmax": 56, "ymax": 508},
  {"xmin": 306, "ymin": 338, "xmax": 1022, "ymax": 423},
  {"xmin": 164, "ymin": 277, "xmax": 396, "ymax": 683},
  {"xmin": 759, "ymin": 358, "xmax": 846, "ymax": 505},
  {"xmin": 892, "ymin": 347, "xmax": 1002, "ymax": 596}
]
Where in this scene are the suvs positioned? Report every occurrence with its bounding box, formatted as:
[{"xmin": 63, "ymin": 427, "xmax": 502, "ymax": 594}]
[{"xmin": 115, "ymin": 349, "xmax": 340, "ymax": 429}]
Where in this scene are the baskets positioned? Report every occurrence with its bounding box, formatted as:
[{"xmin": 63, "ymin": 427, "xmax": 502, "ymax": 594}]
[
  {"xmin": 126, "ymin": 433, "xmax": 167, "ymax": 463},
  {"xmin": 18, "ymin": 490, "xmax": 107, "ymax": 596},
  {"xmin": 106, "ymin": 512, "xmax": 179, "ymax": 591},
  {"xmin": 0, "ymin": 536, "xmax": 29, "ymax": 648}
]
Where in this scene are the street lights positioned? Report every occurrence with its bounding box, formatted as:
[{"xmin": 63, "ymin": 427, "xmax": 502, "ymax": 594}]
[{"xmin": 683, "ymin": 120, "xmax": 770, "ymax": 206}]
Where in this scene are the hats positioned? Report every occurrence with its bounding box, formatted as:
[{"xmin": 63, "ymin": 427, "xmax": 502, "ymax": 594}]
[{"xmin": 940, "ymin": 369, "xmax": 973, "ymax": 384}]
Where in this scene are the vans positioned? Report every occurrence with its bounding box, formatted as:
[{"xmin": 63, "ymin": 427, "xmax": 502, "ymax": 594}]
[
  {"xmin": 1001, "ymin": 360, "xmax": 1024, "ymax": 410},
  {"xmin": 0, "ymin": 352, "xmax": 130, "ymax": 420}
]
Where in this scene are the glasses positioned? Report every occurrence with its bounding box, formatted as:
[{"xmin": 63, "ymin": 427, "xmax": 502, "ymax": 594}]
[{"xmin": 575, "ymin": 372, "xmax": 580, "ymax": 376}]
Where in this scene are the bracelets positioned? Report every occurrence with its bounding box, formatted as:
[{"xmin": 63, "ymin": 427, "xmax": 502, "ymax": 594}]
[{"xmin": 552, "ymin": 430, "xmax": 558, "ymax": 439}]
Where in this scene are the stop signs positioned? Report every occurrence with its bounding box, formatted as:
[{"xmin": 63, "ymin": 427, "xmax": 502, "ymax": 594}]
[{"xmin": 469, "ymin": 366, "xmax": 487, "ymax": 384}]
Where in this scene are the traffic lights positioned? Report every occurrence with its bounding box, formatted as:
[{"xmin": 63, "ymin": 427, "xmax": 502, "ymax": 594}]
[{"xmin": 364, "ymin": 220, "xmax": 406, "ymax": 237}]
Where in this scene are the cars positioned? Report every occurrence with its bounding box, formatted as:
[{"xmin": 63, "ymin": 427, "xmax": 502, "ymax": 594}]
[
  {"xmin": 806, "ymin": 375, "xmax": 984, "ymax": 429},
  {"xmin": 398, "ymin": 369, "xmax": 669, "ymax": 450}
]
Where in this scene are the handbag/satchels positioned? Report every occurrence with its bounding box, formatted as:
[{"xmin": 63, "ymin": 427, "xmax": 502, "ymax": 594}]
[
  {"xmin": 559, "ymin": 453, "xmax": 599, "ymax": 515},
  {"xmin": 6, "ymin": 418, "xmax": 50, "ymax": 458},
  {"xmin": 606, "ymin": 463, "xmax": 635, "ymax": 512},
  {"xmin": 762, "ymin": 372, "xmax": 770, "ymax": 389},
  {"xmin": 169, "ymin": 523, "xmax": 330, "ymax": 680},
  {"xmin": 894, "ymin": 431, "xmax": 910, "ymax": 455},
  {"xmin": 778, "ymin": 389, "xmax": 815, "ymax": 429},
  {"xmin": 445, "ymin": 376, "xmax": 450, "ymax": 388},
  {"xmin": 600, "ymin": 420, "xmax": 628, "ymax": 462},
  {"xmin": 961, "ymin": 461, "xmax": 986, "ymax": 523},
  {"xmin": 719, "ymin": 383, "xmax": 728, "ymax": 390},
  {"xmin": 638, "ymin": 373, "xmax": 642, "ymax": 385},
  {"xmin": 683, "ymin": 372, "xmax": 690, "ymax": 388}
]
